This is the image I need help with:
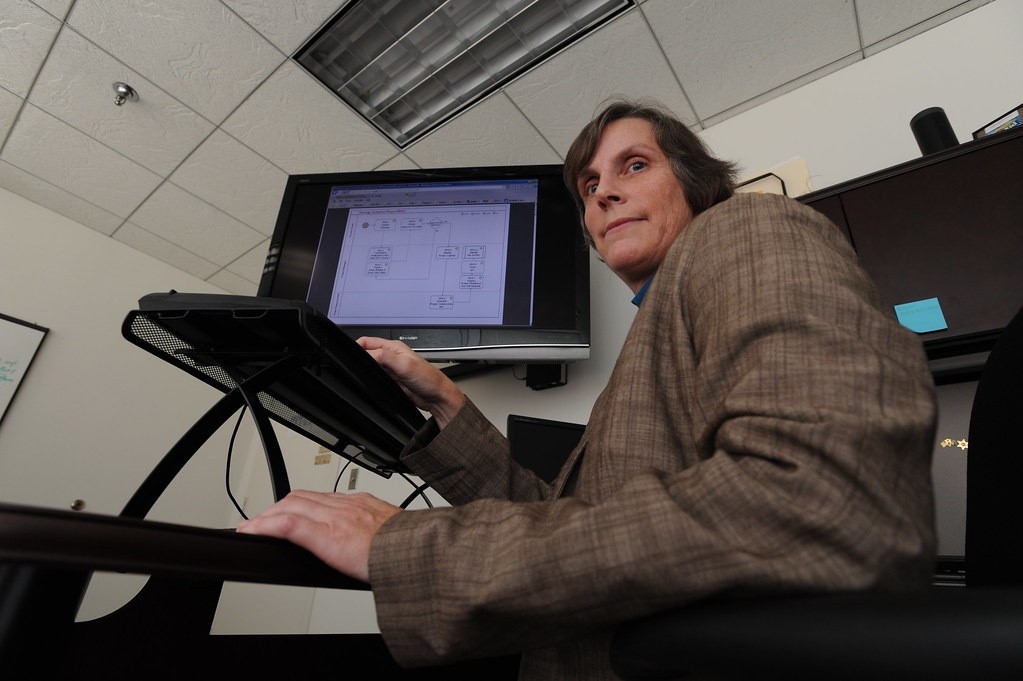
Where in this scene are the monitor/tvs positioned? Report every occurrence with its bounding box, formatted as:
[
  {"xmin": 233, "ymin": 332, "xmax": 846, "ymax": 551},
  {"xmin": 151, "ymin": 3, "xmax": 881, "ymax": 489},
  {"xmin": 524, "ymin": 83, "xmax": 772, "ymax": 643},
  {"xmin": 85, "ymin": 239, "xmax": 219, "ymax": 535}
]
[
  {"xmin": 255, "ymin": 163, "xmax": 590, "ymax": 360},
  {"xmin": 505, "ymin": 415, "xmax": 587, "ymax": 486}
]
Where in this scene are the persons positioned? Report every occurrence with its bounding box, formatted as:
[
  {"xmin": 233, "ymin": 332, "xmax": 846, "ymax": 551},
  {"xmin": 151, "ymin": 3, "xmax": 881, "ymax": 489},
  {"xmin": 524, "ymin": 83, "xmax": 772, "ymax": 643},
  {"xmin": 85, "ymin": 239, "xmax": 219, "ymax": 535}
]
[{"xmin": 236, "ymin": 101, "xmax": 937, "ymax": 681}]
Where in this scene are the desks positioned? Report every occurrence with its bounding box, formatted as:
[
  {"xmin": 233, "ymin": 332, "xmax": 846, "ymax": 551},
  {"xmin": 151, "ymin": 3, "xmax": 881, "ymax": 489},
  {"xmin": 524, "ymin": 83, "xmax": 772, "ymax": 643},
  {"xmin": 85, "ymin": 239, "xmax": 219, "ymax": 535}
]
[{"xmin": 0, "ymin": 502, "xmax": 402, "ymax": 681}]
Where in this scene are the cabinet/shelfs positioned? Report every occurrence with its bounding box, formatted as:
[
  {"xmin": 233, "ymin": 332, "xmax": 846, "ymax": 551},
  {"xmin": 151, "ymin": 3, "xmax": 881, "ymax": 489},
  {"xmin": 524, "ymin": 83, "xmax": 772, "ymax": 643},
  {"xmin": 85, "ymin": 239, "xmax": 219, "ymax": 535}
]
[{"xmin": 792, "ymin": 124, "xmax": 1023, "ymax": 382}]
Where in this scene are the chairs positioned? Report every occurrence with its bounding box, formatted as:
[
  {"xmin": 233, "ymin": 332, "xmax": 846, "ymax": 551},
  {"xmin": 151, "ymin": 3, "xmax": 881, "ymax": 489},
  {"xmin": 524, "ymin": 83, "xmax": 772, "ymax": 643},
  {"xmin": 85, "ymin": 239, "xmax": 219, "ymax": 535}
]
[{"xmin": 611, "ymin": 310, "xmax": 1022, "ymax": 681}]
